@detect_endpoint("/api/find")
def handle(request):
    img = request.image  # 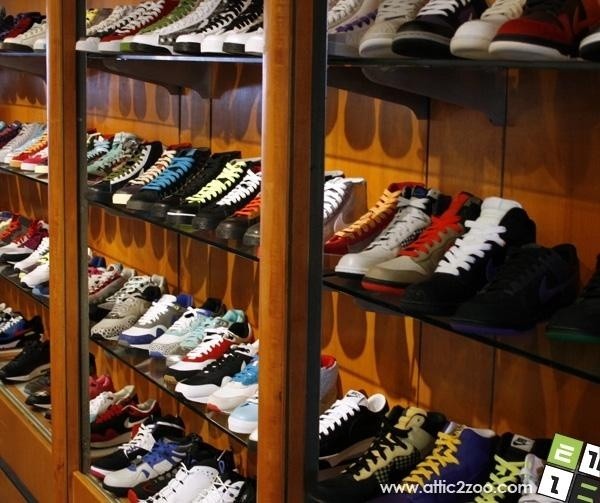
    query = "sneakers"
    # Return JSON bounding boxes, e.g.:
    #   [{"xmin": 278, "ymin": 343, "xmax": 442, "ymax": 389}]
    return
[
  {"xmin": 243, "ymin": 170, "xmax": 344, "ymax": 246},
  {"xmin": 221, "ymin": 20, "xmax": 263, "ymax": 58},
  {"xmin": 88, "ymin": 140, "xmax": 164, "ymax": 203},
  {"xmin": 371, "ymin": 421, "xmax": 500, "ymax": 503},
  {"xmin": 149, "ymin": 298, "xmax": 228, "ymax": 360},
  {"xmin": 489, "ymin": 0, "xmax": 585, "ymax": 63},
  {"xmin": 327, "ymin": 9, "xmax": 379, "ymax": 59},
  {"xmin": 0, "ymin": 302, "xmax": 25, "ymax": 332},
  {"xmin": 25, "ymin": 384, "xmax": 52, "ymax": 409},
  {"xmin": 207, "ymin": 353, "xmax": 260, "ymax": 416},
  {"xmin": 334, "ymin": 185, "xmax": 452, "ymax": 279},
  {"xmin": 0, "ymin": 120, "xmax": 22, "ymax": 150},
  {"xmin": 88, "ymin": 137, "xmax": 149, "ymax": 186},
  {"xmin": 0, "ymin": 17, "xmax": 17, "ymax": 39},
  {"xmin": 35, "ymin": 132, "xmax": 102, "ymax": 175},
  {"xmin": 360, "ymin": 191, "xmax": 482, "ymax": 297},
  {"xmin": 193, "ymin": 460, "xmax": 256, "ymax": 503},
  {"xmin": 118, "ymin": 294, "xmax": 195, "ymax": 351},
  {"xmin": 20, "ymin": 128, "xmax": 97, "ymax": 171},
  {"xmin": 44, "ymin": 374, "xmax": 116, "ymax": 420},
  {"xmin": 0, "ymin": 210, "xmax": 14, "ymax": 232},
  {"xmin": 128, "ymin": 454, "xmax": 188, "ymax": 503},
  {"xmin": 200, "ymin": 0, "xmax": 263, "ymax": 56},
  {"xmin": 32, "ymin": 256, "xmax": 106, "ymax": 300},
  {"xmin": 0, "ymin": 339, "xmax": 51, "ymax": 383},
  {"xmin": 174, "ymin": 0, "xmax": 248, "ymax": 56},
  {"xmin": 215, "ymin": 191, "xmax": 263, "ymax": 239},
  {"xmin": 112, "ymin": 143, "xmax": 192, "ymax": 205},
  {"xmin": 84, "ymin": 5, "xmax": 133, "ymax": 37},
  {"xmin": 167, "ymin": 157, "xmax": 261, "ymax": 225},
  {"xmin": 174, "ymin": 339, "xmax": 259, "ymax": 404},
  {"xmin": 125, "ymin": 147, "xmax": 211, "ymax": 214},
  {"xmin": 191, "ymin": 165, "xmax": 261, "ymax": 231},
  {"xmin": 129, "ymin": 0, "xmax": 219, "ymax": 54},
  {"xmin": 3, "ymin": 17, "xmax": 48, "ymax": 53},
  {"xmin": 319, "ymin": 390, "xmax": 390, "ymax": 469},
  {"xmin": 88, "ymin": 268, "xmax": 138, "ymax": 305},
  {"xmin": 98, "ymin": 1, "xmax": 178, "ymax": 51},
  {"xmin": 1, "ymin": 315, "xmax": 44, "ymax": 350},
  {"xmin": 0, "ymin": 123, "xmax": 40, "ymax": 164},
  {"xmin": 519, "ymin": 454, "xmax": 566, "ymax": 503},
  {"xmin": 88, "ymin": 131, "xmax": 138, "ymax": 178},
  {"xmin": 89, "ymin": 384, "xmax": 139, "ymax": 424},
  {"xmin": 450, "ymin": 433, "xmax": 552, "ymax": 503},
  {"xmin": 325, "ymin": 1, "xmax": 361, "ymax": 27},
  {"xmin": 324, "ymin": 176, "xmax": 368, "ymax": 225},
  {"xmin": 137, "ymin": 441, "xmax": 235, "ymax": 503},
  {"xmin": 163, "ymin": 321, "xmax": 254, "ymax": 386},
  {"xmin": 11, "ymin": 133, "xmax": 48, "ymax": 168},
  {"xmin": 4, "ymin": 122, "xmax": 48, "ymax": 165},
  {"xmin": 309, "ymin": 405, "xmax": 449, "ymax": 502},
  {"xmin": 324, "ymin": 181, "xmax": 425, "ymax": 256},
  {"xmin": 455, "ymin": 243, "xmax": 581, "ymax": 337},
  {"xmin": 247, "ymin": 355, "xmax": 339, "ymax": 451},
  {"xmin": 399, "ymin": 197, "xmax": 536, "ymax": 317},
  {"xmin": 87, "ymin": 263, "xmax": 122, "ymax": 288},
  {"xmin": 86, "ymin": 133, "xmax": 114, "ymax": 165},
  {"xmin": 0, "ymin": 215, "xmax": 49, "ymax": 266},
  {"xmin": 0, "ymin": 11, "xmax": 45, "ymax": 51},
  {"xmin": 572, "ymin": 1, "xmax": 600, "ymax": 62},
  {"xmin": 23, "ymin": 353, "xmax": 98, "ymax": 395},
  {"xmin": 0, "ymin": 213, "xmax": 33, "ymax": 246},
  {"xmin": 89, "ymin": 397, "xmax": 161, "ymax": 449},
  {"xmin": 151, "ymin": 151, "xmax": 241, "ymax": 218},
  {"xmin": 544, "ymin": 253, "xmax": 599, "ymax": 345},
  {"xmin": 88, "ymin": 274, "xmax": 169, "ymax": 321},
  {"xmin": 103, "ymin": 432, "xmax": 203, "ymax": 496},
  {"xmin": 166, "ymin": 308, "xmax": 248, "ymax": 367},
  {"xmin": 18, "ymin": 251, "xmax": 50, "ymax": 279},
  {"xmin": 76, "ymin": 1, "xmax": 150, "ymax": 49},
  {"xmin": 358, "ymin": 1, "xmax": 426, "ymax": 59},
  {"xmin": 89, "ymin": 414, "xmax": 186, "ymax": 478},
  {"xmin": 20, "ymin": 248, "xmax": 94, "ymax": 290},
  {"xmin": 449, "ymin": 0, "xmax": 526, "ymax": 61},
  {"xmin": 227, "ymin": 386, "xmax": 262, "ymax": 435},
  {"xmin": 392, "ymin": 0, "xmax": 489, "ymax": 59},
  {"xmin": 14, "ymin": 237, "xmax": 49, "ymax": 274},
  {"xmin": 119, "ymin": 1, "xmax": 195, "ymax": 53},
  {"xmin": 89, "ymin": 286, "xmax": 162, "ymax": 341}
]
